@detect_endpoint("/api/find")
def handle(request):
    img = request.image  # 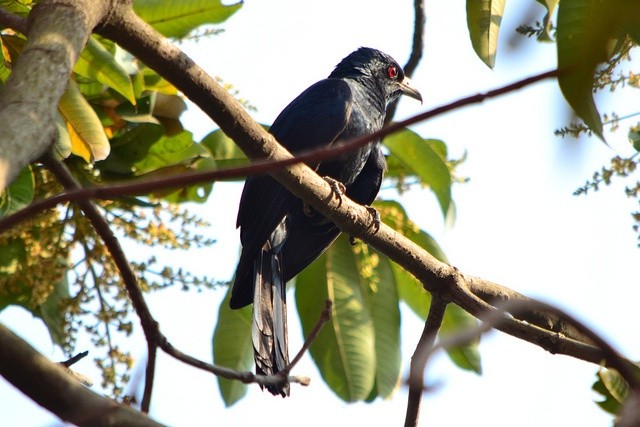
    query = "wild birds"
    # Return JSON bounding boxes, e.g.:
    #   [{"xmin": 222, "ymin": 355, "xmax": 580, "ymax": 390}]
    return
[{"xmin": 228, "ymin": 44, "xmax": 425, "ymax": 400}]
